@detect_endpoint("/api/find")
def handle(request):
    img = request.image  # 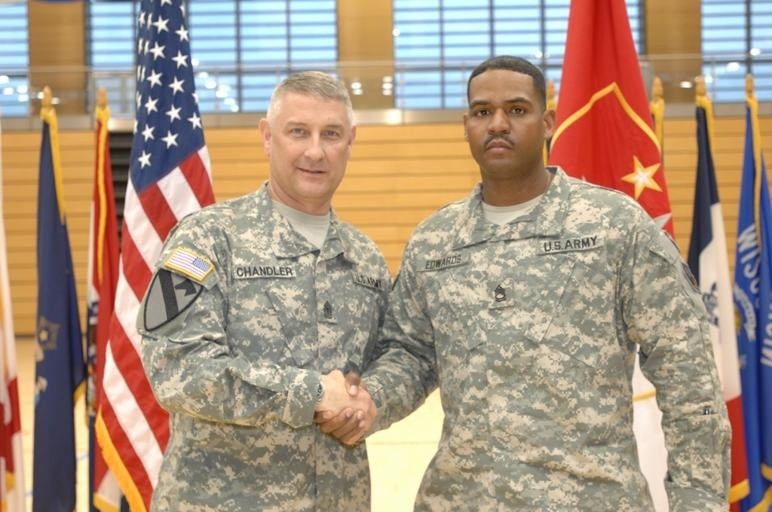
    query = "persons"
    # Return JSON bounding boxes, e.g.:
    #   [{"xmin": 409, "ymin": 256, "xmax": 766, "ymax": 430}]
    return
[
  {"xmin": 312, "ymin": 57, "xmax": 733, "ymax": 512},
  {"xmin": 136, "ymin": 71, "xmax": 393, "ymax": 512}
]
